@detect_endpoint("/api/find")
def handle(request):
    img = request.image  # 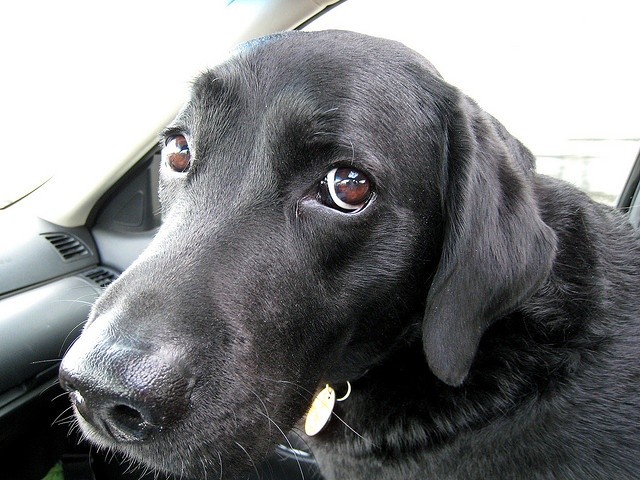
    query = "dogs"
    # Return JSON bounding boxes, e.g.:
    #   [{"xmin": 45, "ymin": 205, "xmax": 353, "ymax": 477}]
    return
[{"xmin": 59, "ymin": 29, "xmax": 640, "ymax": 480}]
